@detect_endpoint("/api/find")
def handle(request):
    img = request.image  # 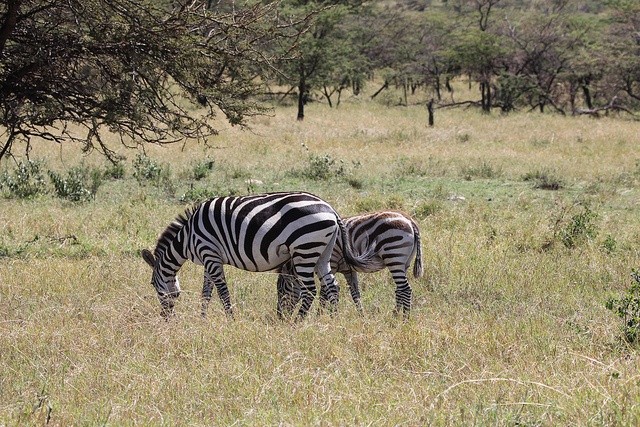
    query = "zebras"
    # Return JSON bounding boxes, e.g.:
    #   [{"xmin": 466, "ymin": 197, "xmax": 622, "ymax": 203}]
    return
[
  {"xmin": 277, "ymin": 210, "xmax": 424, "ymax": 322},
  {"xmin": 141, "ymin": 191, "xmax": 382, "ymax": 322}
]
